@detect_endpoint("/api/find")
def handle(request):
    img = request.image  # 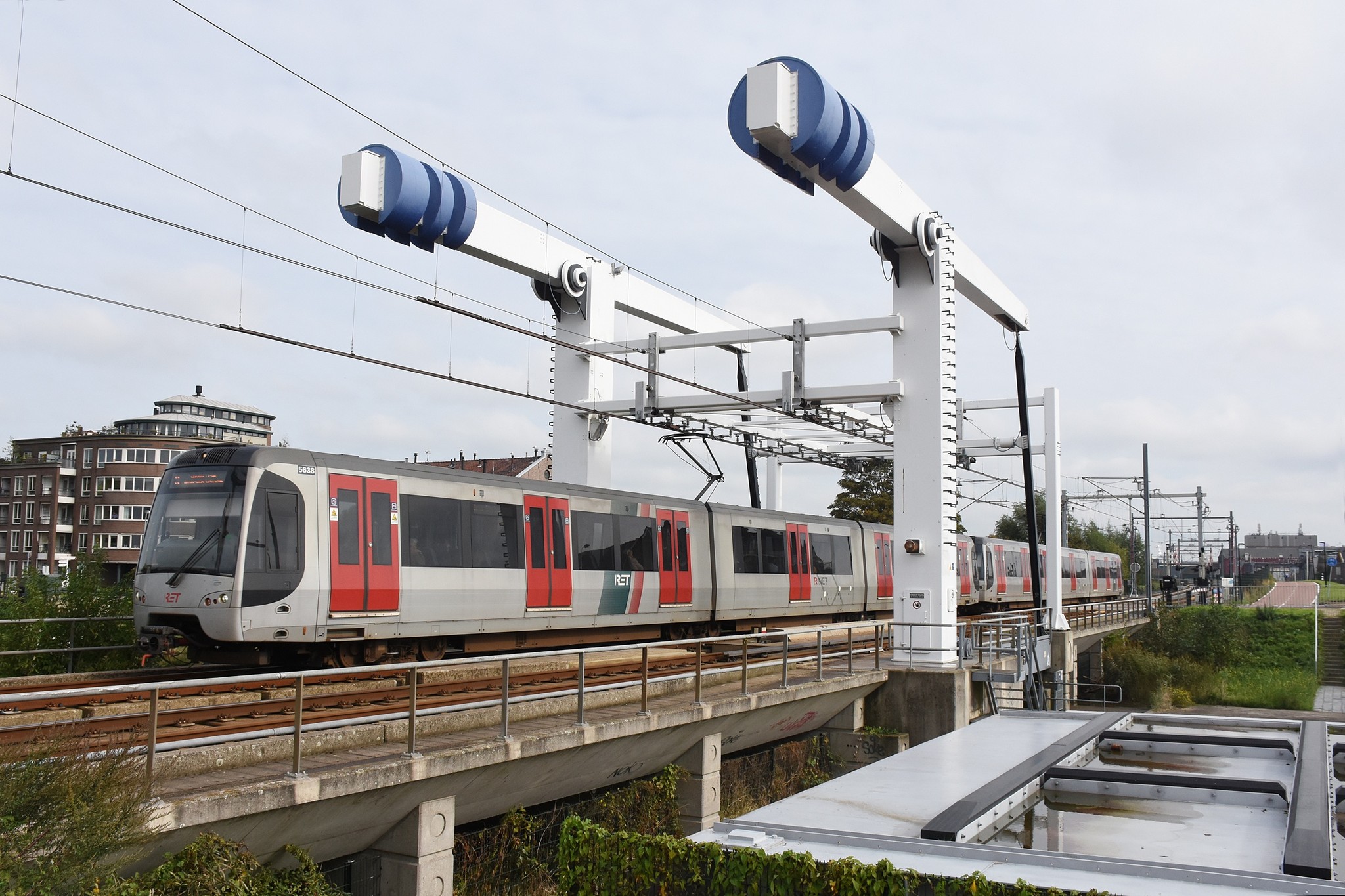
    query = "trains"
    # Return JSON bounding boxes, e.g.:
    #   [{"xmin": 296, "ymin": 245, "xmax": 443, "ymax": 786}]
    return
[{"xmin": 131, "ymin": 442, "xmax": 1124, "ymax": 672}]
